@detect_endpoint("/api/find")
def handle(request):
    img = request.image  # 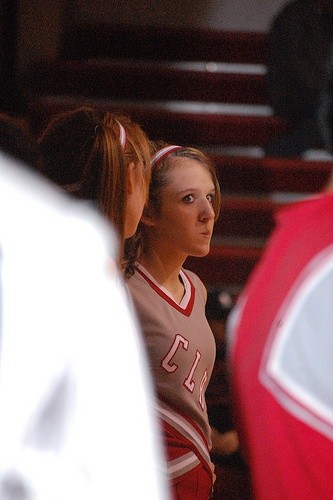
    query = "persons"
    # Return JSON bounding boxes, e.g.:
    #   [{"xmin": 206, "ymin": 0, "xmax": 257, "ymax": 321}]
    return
[
  {"xmin": 29, "ymin": 106, "xmax": 152, "ymax": 268},
  {"xmin": 0, "ymin": 150, "xmax": 171, "ymax": 500},
  {"xmin": 224, "ymin": 56, "xmax": 333, "ymax": 500},
  {"xmin": 118, "ymin": 139, "xmax": 217, "ymax": 500},
  {"xmin": 205, "ymin": 293, "xmax": 255, "ymax": 500}
]
